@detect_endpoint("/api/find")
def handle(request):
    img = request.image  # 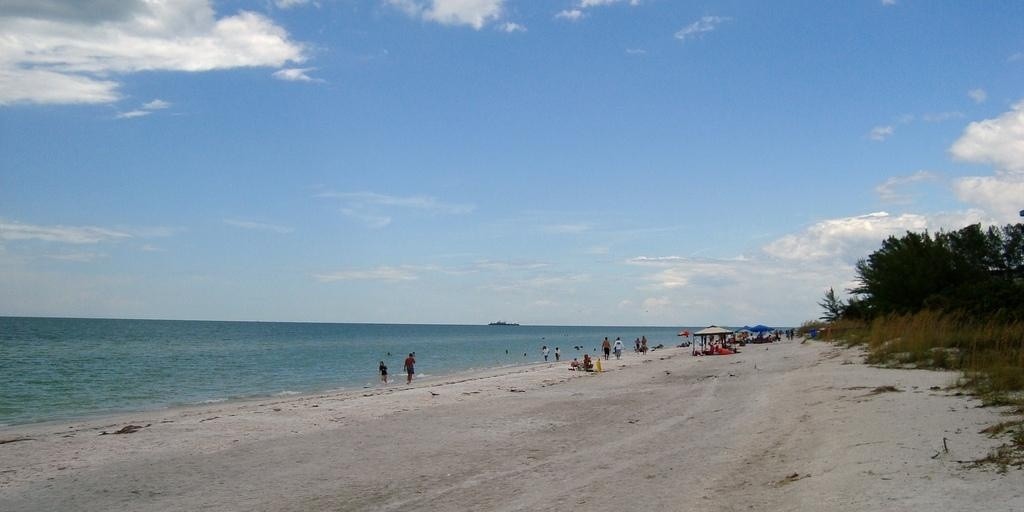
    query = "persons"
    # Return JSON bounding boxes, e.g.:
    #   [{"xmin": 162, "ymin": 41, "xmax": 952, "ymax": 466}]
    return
[
  {"xmin": 675, "ymin": 322, "xmax": 796, "ymax": 356},
  {"xmin": 538, "ymin": 334, "xmax": 664, "ymax": 373},
  {"xmin": 402, "ymin": 352, "xmax": 415, "ymax": 386},
  {"xmin": 377, "ymin": 361, "xmax": 388, "ymax": 386}
]
